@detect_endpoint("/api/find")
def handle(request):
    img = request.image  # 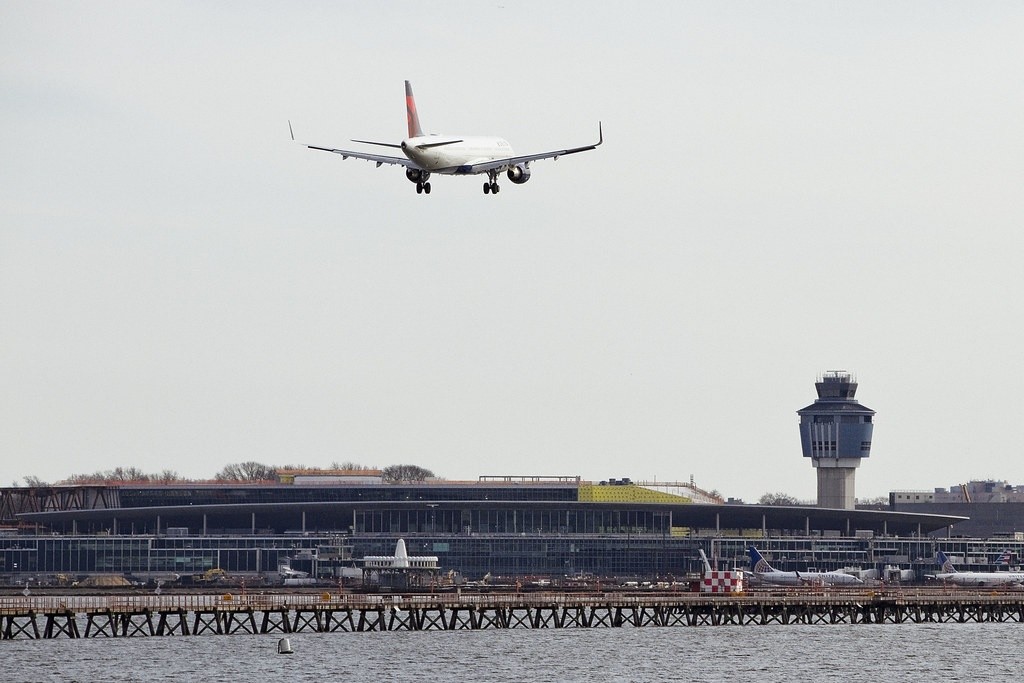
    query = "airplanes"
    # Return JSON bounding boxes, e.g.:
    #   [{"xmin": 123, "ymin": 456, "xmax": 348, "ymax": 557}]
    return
[
  {"xmin": 924, "ymin": 551, "xmax": 1024, "ymax": 589},
  {"xmin": 733, "ymin": 545, "xmax": 864, "ymax": 587},
  {"xmin": 286, "ymin": 80, "xmax": 603, "ymax": 194}
]
